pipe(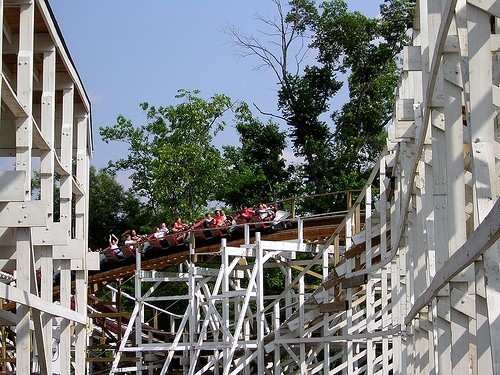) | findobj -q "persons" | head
[89,202,279,264]
